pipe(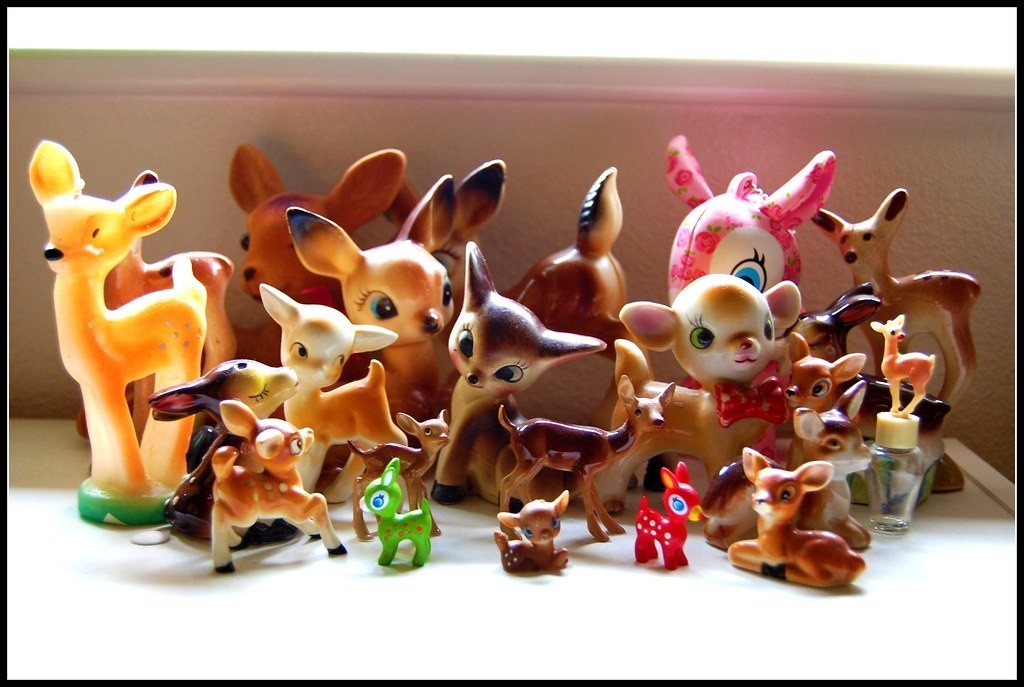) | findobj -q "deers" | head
[24,135,980,589]
